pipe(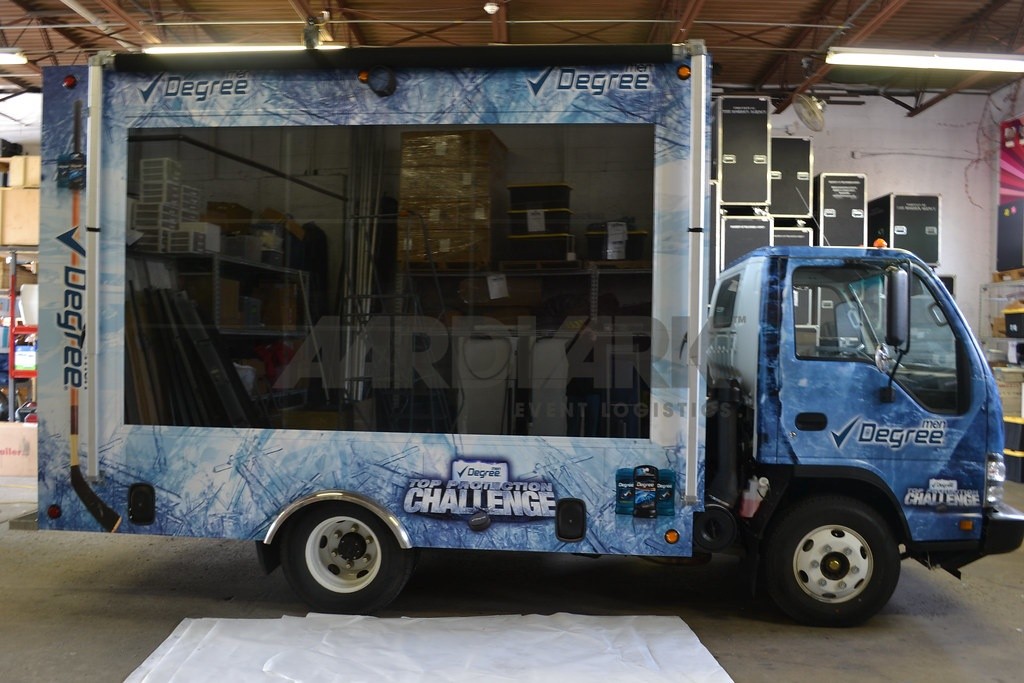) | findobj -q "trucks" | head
[37,38,1024,625]
